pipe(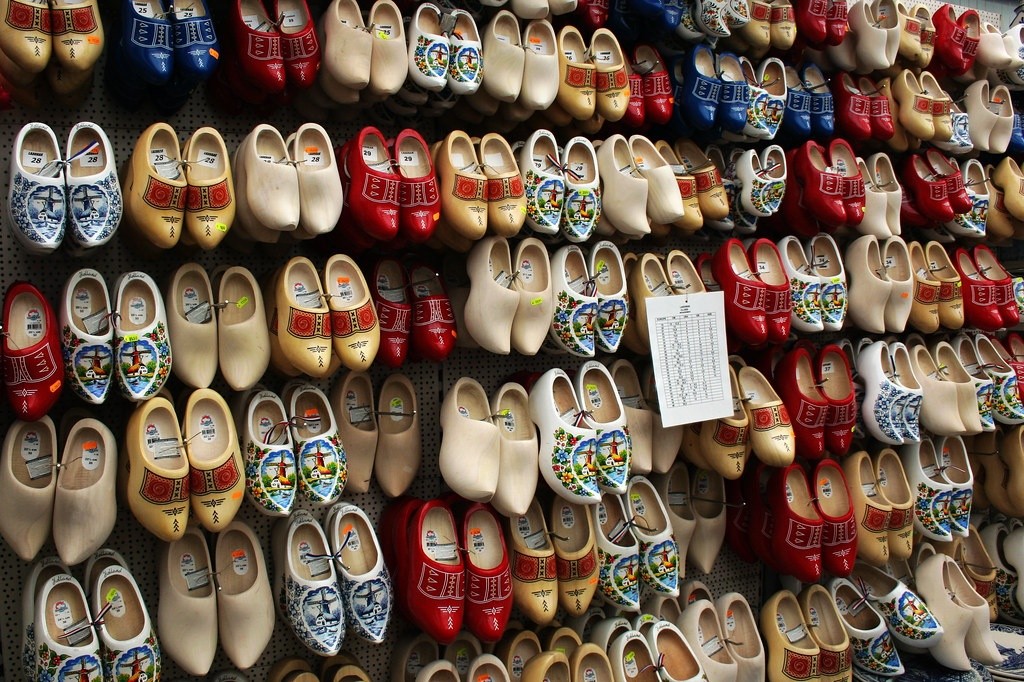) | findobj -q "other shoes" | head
[0,0,1024,682]
